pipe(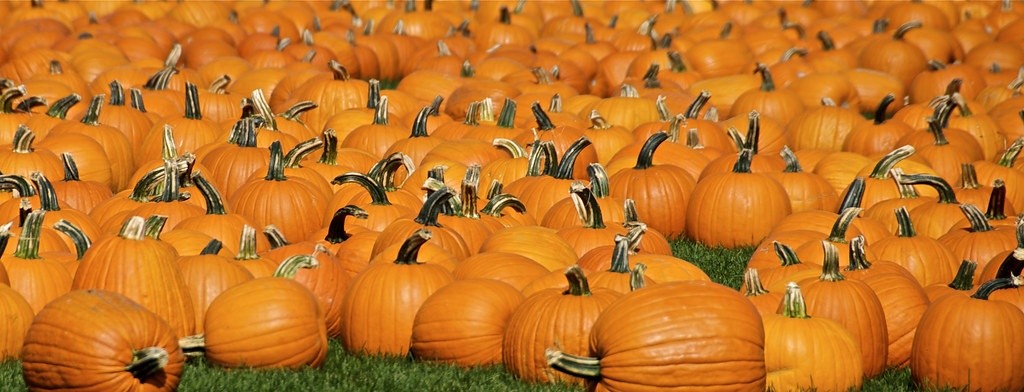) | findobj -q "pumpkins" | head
[0,0,1024,392]
